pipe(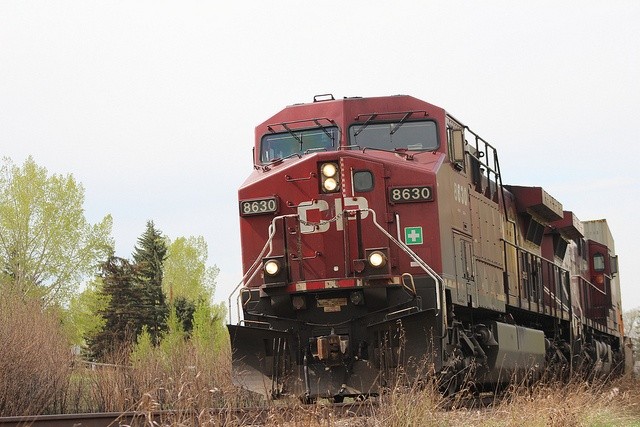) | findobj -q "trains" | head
[225,93,634,405]
